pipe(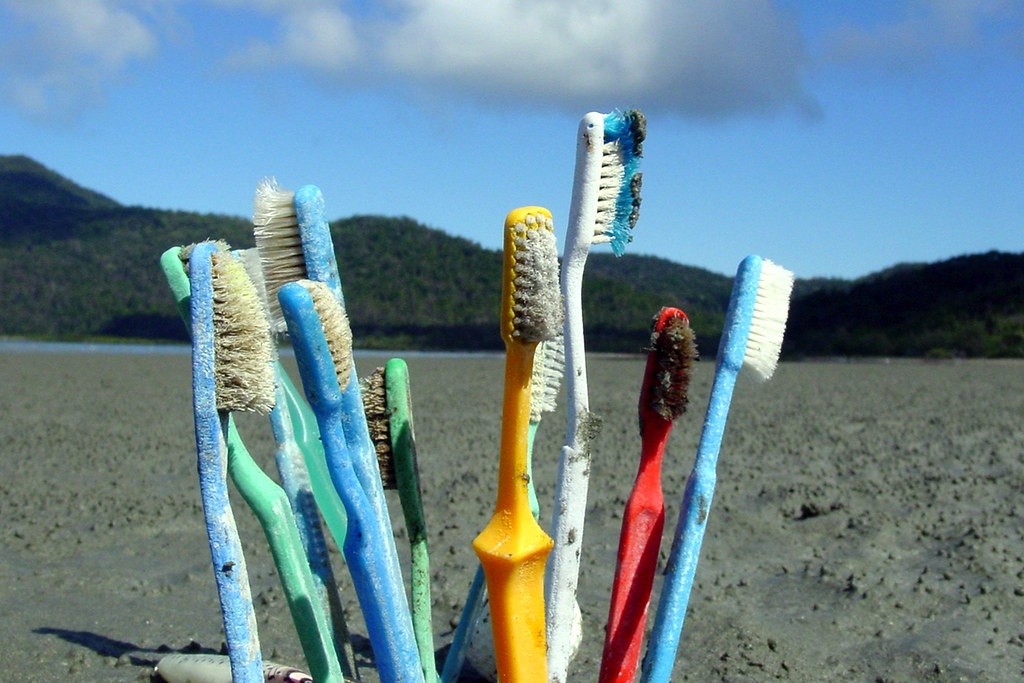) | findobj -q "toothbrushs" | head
[230,246,362,683]
[472,205,564,681]
[276,274,431,683]
[598,305,698,683]
[440,332,568,683]
[153,653,315,683]
[643,250,795,681]
[362,358,441,683]
[159,242,349,683]
[189,238,278,683]
[251,174,431,683]
[547,103,647,680]
